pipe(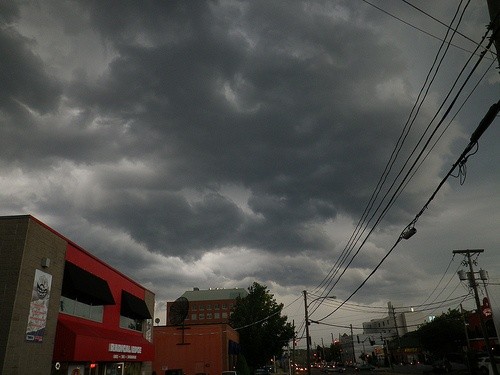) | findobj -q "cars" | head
[290,361,339,372]
